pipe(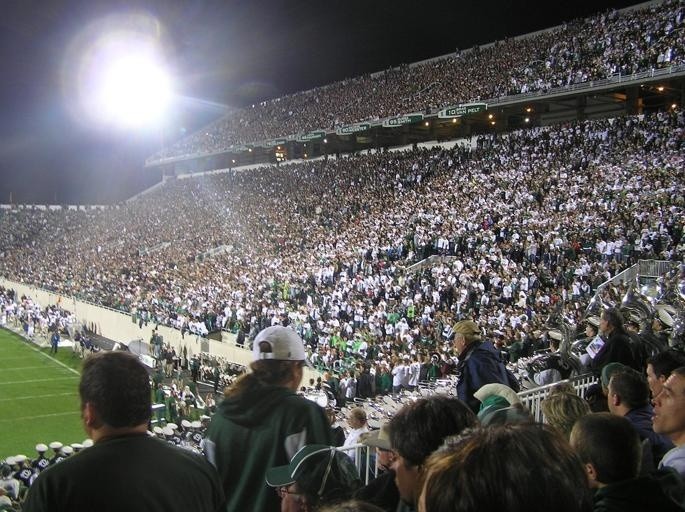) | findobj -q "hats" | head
[3,436,97,467]
[657,307,674,329]
[152,413,212,437]
[362,423,394,452]
[452,320,482,336]
[248,323,308,363]
[264,445,364,494]
[587,317,600,327]
